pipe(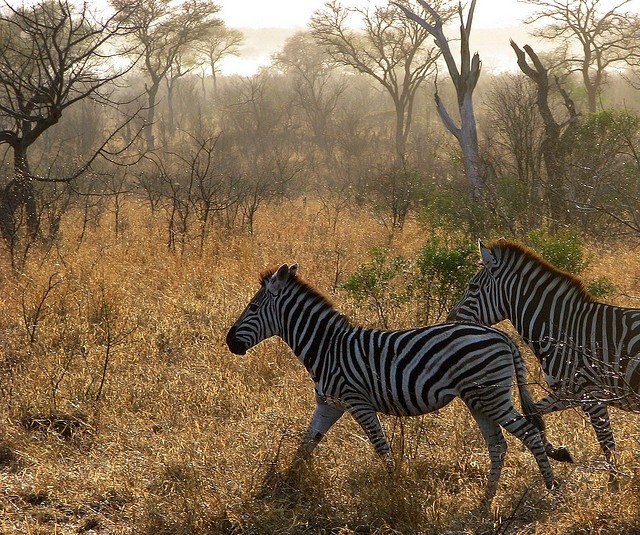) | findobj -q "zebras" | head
[224,262,553,514]
[445,235,640,492]
